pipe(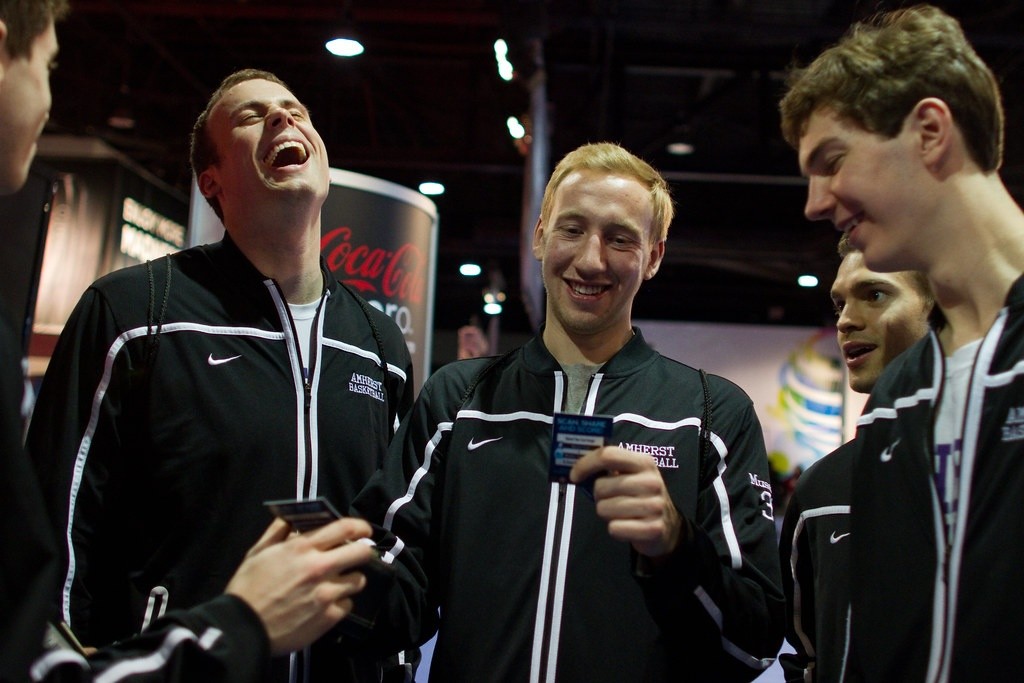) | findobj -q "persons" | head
[288,141,788,683]
[777,5,1024,683]
[777,231,934,683]
[0,1,379,683]
[23,67,441,683]
